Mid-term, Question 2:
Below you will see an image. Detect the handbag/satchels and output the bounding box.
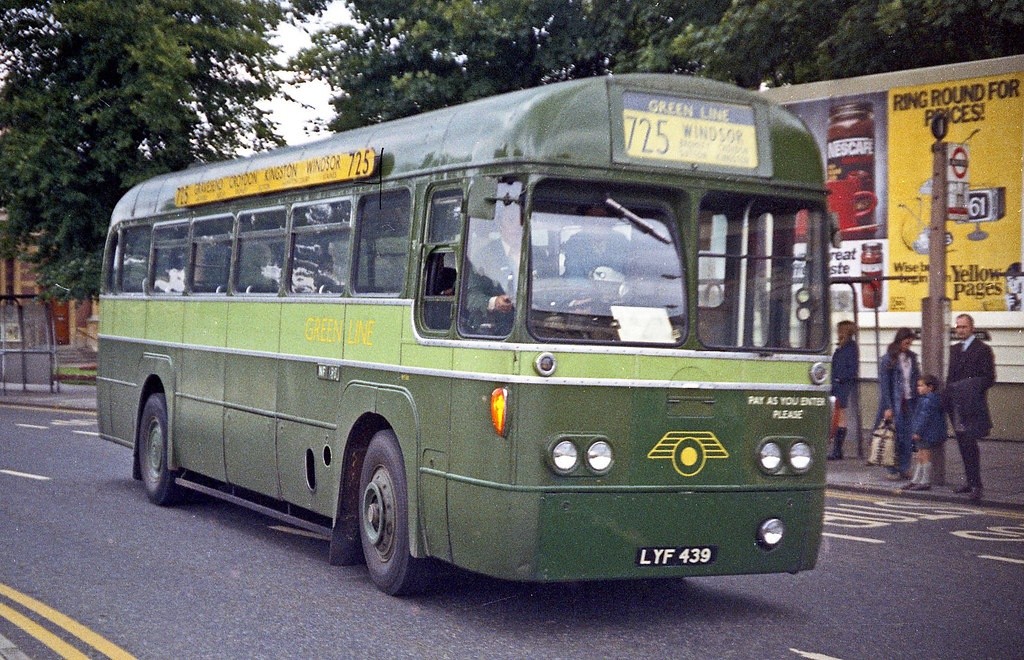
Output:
[867,416,896,467]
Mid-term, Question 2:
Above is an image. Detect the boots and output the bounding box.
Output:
[827,427,848,460]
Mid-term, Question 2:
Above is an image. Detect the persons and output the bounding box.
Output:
[563,203,629,277]
[899,375,948,489]
[827,321,859,460]
[877,328,920,487]
[944,314,995,501]
[463,201,545,331]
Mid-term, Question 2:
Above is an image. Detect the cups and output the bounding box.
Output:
[824,180,878,227]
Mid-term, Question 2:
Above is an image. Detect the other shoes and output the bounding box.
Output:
[885,469,912,481]
[910,483,930,491]
[900,482,916,490]
[953,485,970,494]
[969,487,982,500]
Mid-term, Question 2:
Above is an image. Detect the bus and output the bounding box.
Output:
[98,71,842,599]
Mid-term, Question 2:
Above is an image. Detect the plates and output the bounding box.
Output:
[839,224,877,240]
